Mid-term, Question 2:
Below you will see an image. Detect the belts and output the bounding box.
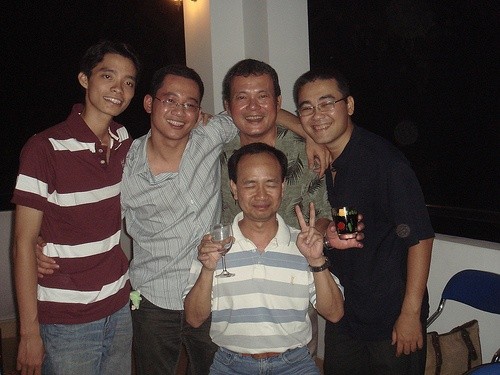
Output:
[239,350,294,360]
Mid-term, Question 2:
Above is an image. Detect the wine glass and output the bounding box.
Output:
[210,223,236,279]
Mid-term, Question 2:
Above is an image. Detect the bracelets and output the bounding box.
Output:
[322,236,333,250]
[308,259,328,272]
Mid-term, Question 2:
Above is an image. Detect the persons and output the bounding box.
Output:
[33,64,334,375]
[196,58,364,361]
[182,143,345,375]
[11,41,139,375]
[292,69,436,375]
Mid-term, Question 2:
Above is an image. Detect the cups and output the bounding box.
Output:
[330,205,359,240]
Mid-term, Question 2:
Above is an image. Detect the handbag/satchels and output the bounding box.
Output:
[423,320,482,375]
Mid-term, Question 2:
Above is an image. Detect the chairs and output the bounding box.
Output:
[421,269,500,375]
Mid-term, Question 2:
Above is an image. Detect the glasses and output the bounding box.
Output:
[295,97,345,117]
[152,96,201,111]
